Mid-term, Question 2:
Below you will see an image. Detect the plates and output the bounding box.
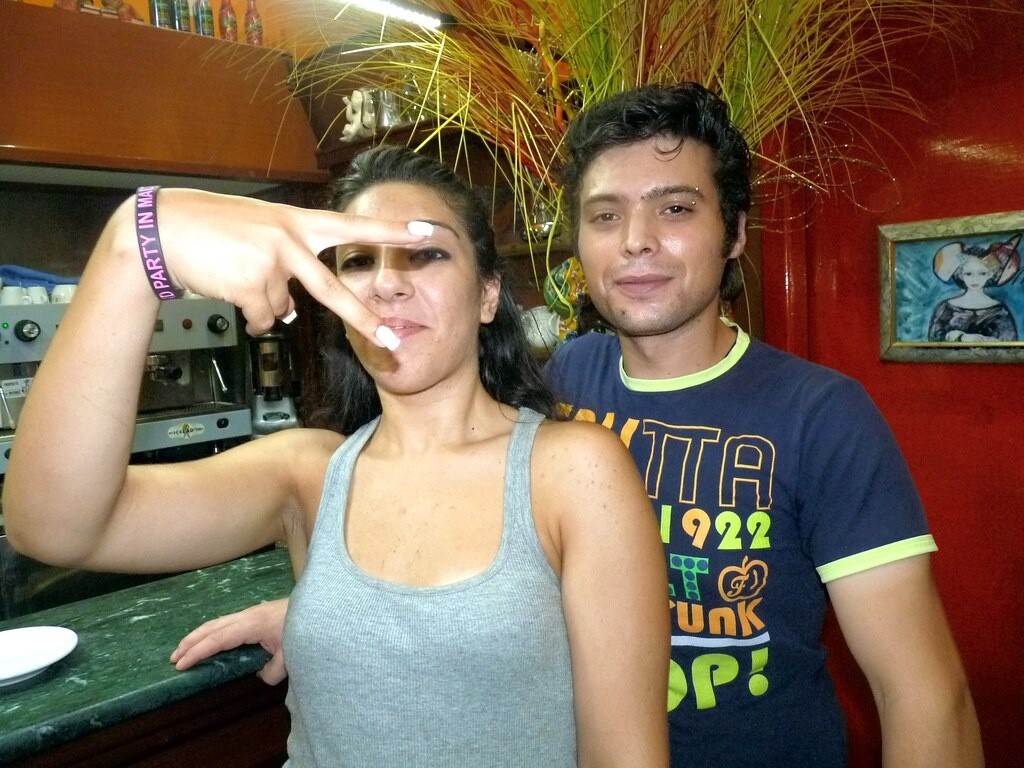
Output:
[0,626,77,686]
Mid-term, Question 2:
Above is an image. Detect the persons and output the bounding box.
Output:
[170,81,987,767]
[3,147,671,768]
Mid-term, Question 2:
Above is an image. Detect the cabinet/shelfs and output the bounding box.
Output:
[312,75,592,259]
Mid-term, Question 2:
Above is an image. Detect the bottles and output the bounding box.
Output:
[192,0,214,37]
[150,0,172,30]
[218,0,237,41]
[244,0,262,46]
[172,0,190,33]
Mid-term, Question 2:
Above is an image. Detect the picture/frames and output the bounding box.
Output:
[876,211,1023,364]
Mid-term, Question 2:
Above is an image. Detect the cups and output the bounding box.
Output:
[0,284,77,305]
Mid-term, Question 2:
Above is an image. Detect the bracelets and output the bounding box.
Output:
[136,184,184,301]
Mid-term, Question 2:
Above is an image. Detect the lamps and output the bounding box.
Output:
[337,0,459,30]
